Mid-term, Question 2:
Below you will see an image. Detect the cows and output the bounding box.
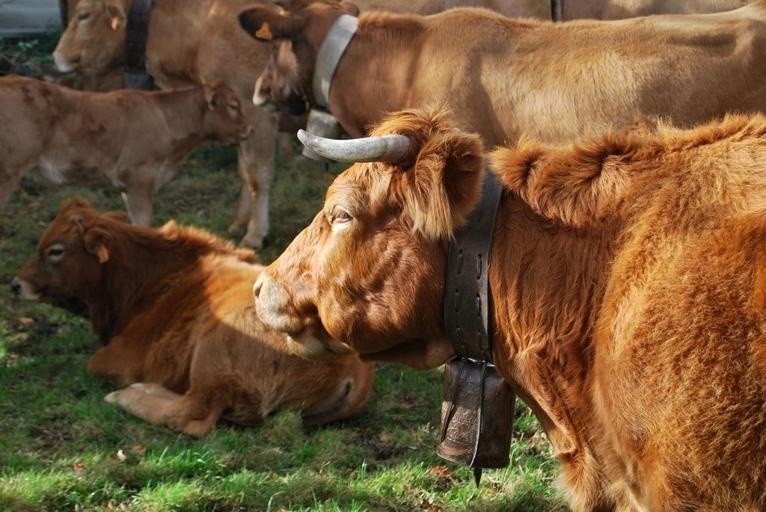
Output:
[0,2,765,512]
